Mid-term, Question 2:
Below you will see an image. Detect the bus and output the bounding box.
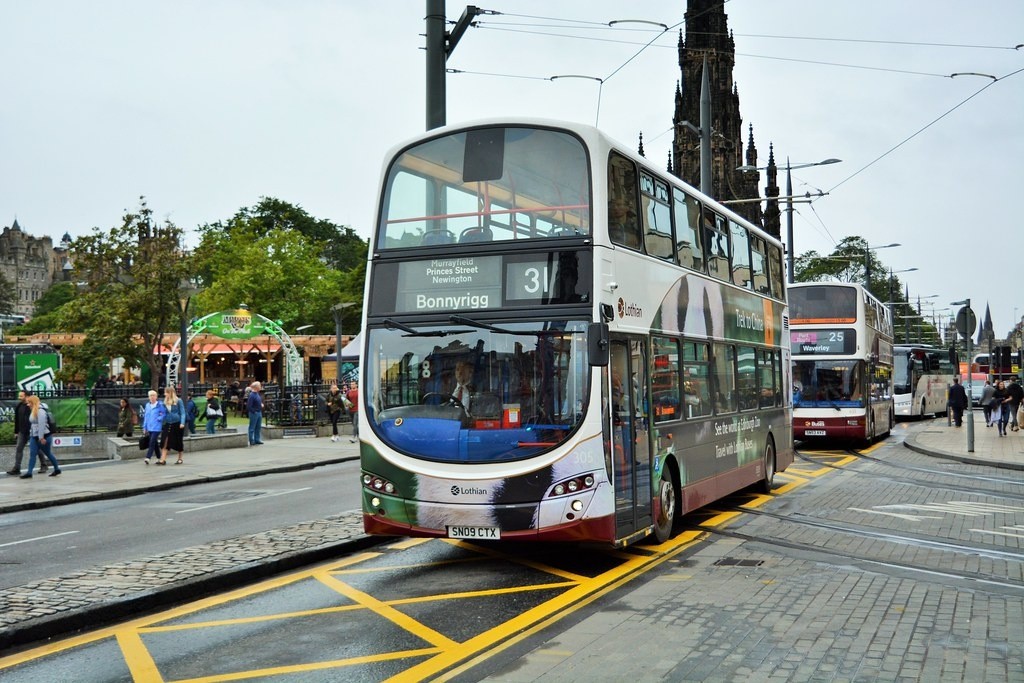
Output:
[788,278,894,448]
[355,115,794,552]
[893,343,961,420]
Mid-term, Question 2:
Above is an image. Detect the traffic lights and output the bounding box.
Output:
[928,353,941,371]
[992,346,1000,367]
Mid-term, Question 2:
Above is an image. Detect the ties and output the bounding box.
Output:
[458,387,463,401]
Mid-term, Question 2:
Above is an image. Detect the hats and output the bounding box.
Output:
[1010,376,1017,381]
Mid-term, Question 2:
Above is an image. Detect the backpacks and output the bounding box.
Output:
[191,405,199,416]
[46,412,57,434]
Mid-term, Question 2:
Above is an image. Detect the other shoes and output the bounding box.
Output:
[635,462,641,465]
[176,459,183,464]
[145,458,149,465]
[349,439,356,443]
[19,474,33,479]
[1009,424,1013,431]
[156,460,166,465]
[255,442,264,444]
[7,469,20,475]
[250,441,255,445]
[38,465,48,473]
[331,436,339,442]
[49,470,60,476]
[1013,428,1019,432]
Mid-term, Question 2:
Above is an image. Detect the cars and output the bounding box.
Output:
[959,351,1024,408]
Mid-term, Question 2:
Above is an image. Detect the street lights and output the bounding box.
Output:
[894,282,940,345]
[843,240,903,295]
[914,305,951,346]
[330,301,357,396]
[870,267,919,336]
[173,285,206,437]
[734,155,843,284]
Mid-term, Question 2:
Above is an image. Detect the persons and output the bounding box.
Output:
[746,384,781,406]
[7,390,61,479]
[978,376,1024,436]
[948,377,968,428]
[440,357,481,418]
[906,354,916,386]
[225,381,264,445]
[117,385,224,465]
[324,381,358,443]
[609,198,640,247]
[608,371,648,466]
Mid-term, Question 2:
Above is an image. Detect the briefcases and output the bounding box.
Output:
[139,433,150,450]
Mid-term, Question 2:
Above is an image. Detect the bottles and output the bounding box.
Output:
[340,397,354,408]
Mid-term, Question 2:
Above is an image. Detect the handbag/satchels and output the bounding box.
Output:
[207,402,224,416]
[132,411,138,424]
[178,399,192,422]
[324,397,330,412]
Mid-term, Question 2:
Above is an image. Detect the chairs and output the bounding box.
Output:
[401,222,580,242]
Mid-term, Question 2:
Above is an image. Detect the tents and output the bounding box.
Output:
[322,327,421,385]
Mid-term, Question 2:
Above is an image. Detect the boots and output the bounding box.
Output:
[1003,422,1007,435]
[998,424,1002,436]
